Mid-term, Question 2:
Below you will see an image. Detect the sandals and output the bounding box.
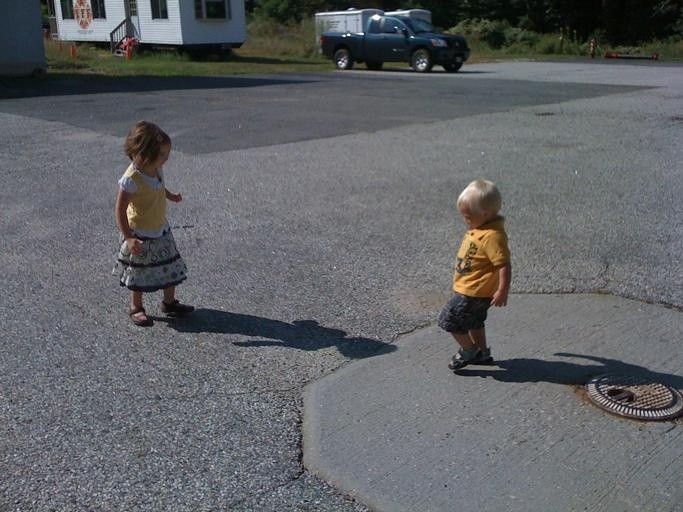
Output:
[447,347,482,370]
[471,347,492,365]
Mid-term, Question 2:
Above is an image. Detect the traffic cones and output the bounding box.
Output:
[57,43,65,53]
[71,41,79,59]
[125,38,133,60]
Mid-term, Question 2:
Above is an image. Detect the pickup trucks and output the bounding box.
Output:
[320,14,471,73]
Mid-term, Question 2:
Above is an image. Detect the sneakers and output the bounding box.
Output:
[161,299,194,313]
[128,305,152,326]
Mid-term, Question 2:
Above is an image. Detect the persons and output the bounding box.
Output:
[438,178,513,369]
[116,121,195,326]
